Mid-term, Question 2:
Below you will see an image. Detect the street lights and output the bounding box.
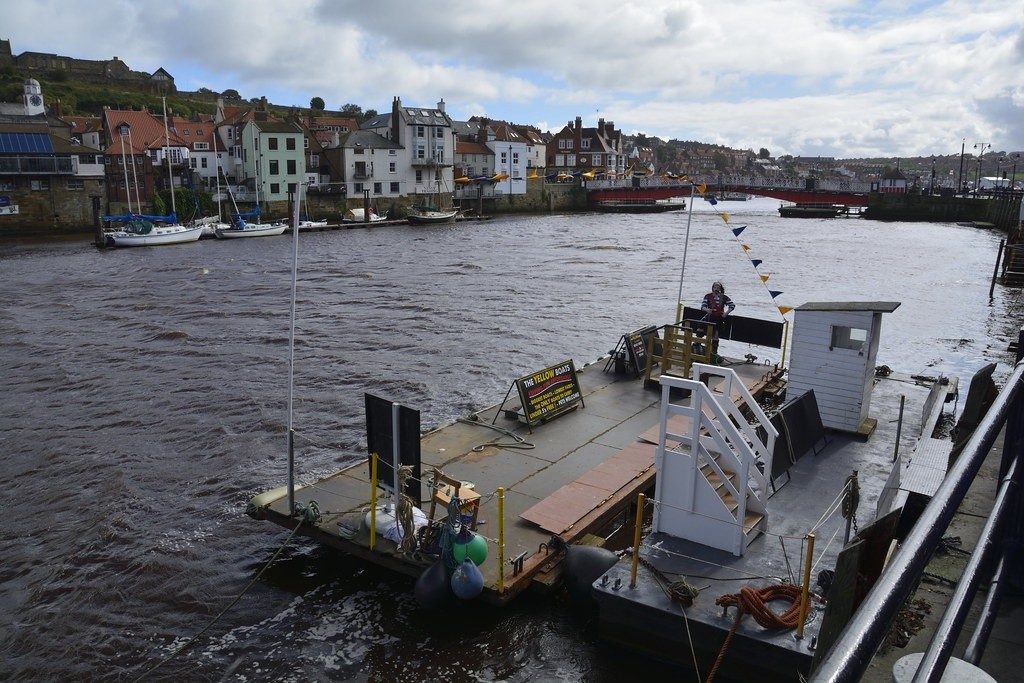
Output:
[1009,154,1020,200]
[994,157,1002,199]
[974,143,991,189]
[930,159,936,195]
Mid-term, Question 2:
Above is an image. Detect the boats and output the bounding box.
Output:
[343,208,387,223]
[778,201,839,218]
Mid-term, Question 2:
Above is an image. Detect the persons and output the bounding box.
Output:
[694,282,735,354]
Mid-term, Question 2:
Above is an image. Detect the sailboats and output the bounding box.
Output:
[407,163,458,223]
[98,96,287,247]
[287,182,328,231]
